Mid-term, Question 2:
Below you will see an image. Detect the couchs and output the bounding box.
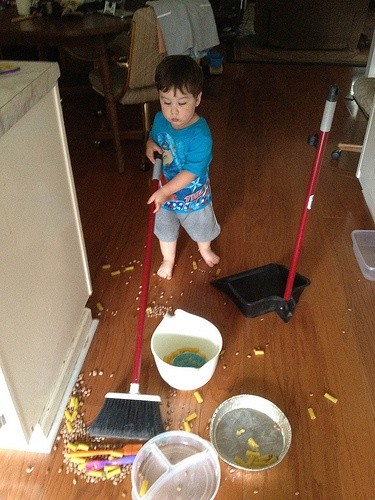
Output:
[253,0,371,49]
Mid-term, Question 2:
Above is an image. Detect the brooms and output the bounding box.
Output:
[85,150,165,441]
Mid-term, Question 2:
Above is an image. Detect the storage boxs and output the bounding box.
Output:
[351,230,375,281]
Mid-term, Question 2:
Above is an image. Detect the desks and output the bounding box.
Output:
[0,9,134,173]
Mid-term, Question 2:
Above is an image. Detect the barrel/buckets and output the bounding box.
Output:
[150,308,222,391]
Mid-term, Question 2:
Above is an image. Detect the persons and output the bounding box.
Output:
[145,56,220,280]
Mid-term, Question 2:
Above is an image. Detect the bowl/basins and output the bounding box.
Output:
[210,394,292,471]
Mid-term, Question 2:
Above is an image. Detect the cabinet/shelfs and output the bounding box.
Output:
[0,61,98,455]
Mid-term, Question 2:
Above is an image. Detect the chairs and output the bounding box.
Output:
[331,76,375,158]
[93,0,220,171]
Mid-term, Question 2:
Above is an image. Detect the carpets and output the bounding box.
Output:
[226,0,375,65]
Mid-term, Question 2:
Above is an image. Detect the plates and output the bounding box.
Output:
[131,431,221,500]
